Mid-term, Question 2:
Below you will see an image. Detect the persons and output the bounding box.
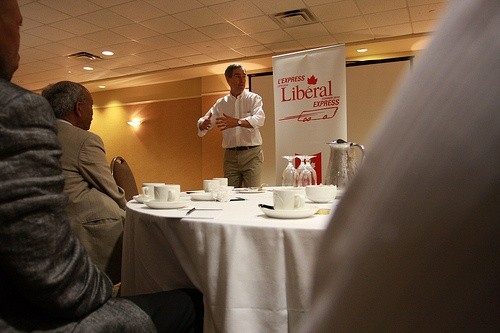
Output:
[41,80,129,285]
[303,1,500,333]
[0,0,206,333]
[197,64,265,187]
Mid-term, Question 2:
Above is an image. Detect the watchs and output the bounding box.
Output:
[237,119,242,126]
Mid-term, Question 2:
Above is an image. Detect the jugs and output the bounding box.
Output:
[324,138,366,190]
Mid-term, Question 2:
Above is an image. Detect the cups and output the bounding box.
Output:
[154,184,181,202]
[273,187,306,210]
[202,177,235,201]
[140,182,165,197]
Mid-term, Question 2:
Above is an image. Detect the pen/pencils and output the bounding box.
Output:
[186,207,196,215]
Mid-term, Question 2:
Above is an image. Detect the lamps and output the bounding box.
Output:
[127,117,146,127]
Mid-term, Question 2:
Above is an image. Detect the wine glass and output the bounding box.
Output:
[281,155,318,187]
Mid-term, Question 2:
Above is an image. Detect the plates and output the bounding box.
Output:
[261,204,322,218]
[190,191,217,200]
[133,194,191,208]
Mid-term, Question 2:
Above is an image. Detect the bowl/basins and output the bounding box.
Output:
[306,184,337,203]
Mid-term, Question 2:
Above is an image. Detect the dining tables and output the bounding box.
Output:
[120,185,348,333]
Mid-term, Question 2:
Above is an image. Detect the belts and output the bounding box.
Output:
[228,146,257,151]
